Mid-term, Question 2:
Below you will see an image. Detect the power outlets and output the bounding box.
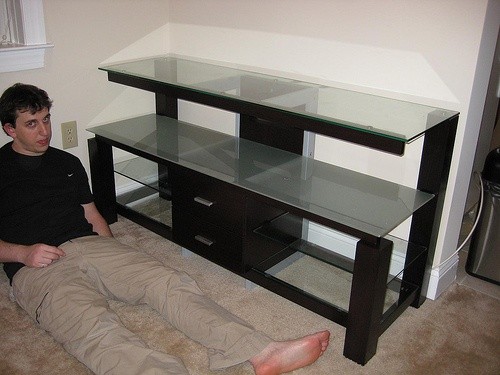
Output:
[60,120,79,150]
[469,158,484,187]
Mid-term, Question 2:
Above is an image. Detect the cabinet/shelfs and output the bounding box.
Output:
[84,54,461,366]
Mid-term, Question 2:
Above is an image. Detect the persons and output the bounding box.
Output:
[0,82,330,375]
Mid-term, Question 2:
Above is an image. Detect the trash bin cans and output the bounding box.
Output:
[465,148,500,286]
